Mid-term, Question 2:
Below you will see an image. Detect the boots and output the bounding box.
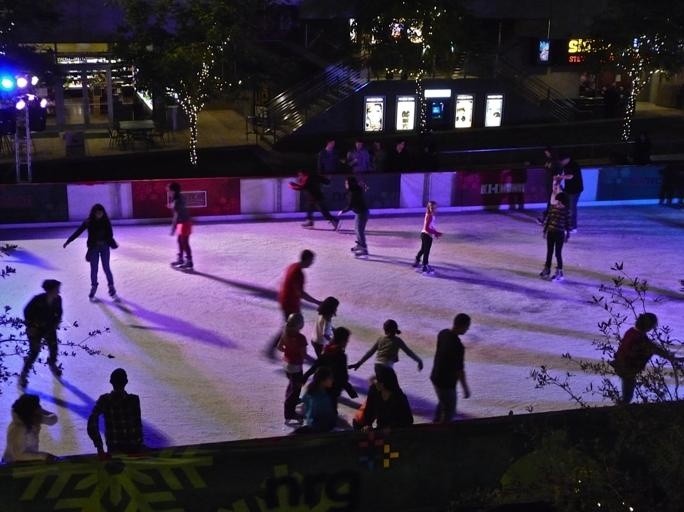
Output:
[413,259,432,272]
[285,408,303,419]
[301,221,313,226]
[332,219,339,231]
[539,265,564,280]
[351,240,367,257]
[170,252,183,266]
[89,282,98,299]
[107,282,115,296]
[181,254,193,269]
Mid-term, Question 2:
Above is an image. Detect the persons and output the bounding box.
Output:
[1,391,59,463]
[86,367,144,455]
[266,249,323,359]
[538,148,585,280]
[338,177,371,256]
[365,104,382,132]
[63,203,117,298]
[165,181,193,269]
[414,200,443,272]
[315,139,406,173]
[17,278,64,388]
[613,312,675,403]
[288,169,339,230]
[275,295,471,432]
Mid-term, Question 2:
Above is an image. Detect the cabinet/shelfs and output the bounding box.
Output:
[56,52,139,96]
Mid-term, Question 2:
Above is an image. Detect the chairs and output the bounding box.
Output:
[107,115,167,151]
[0,119,36,156]
